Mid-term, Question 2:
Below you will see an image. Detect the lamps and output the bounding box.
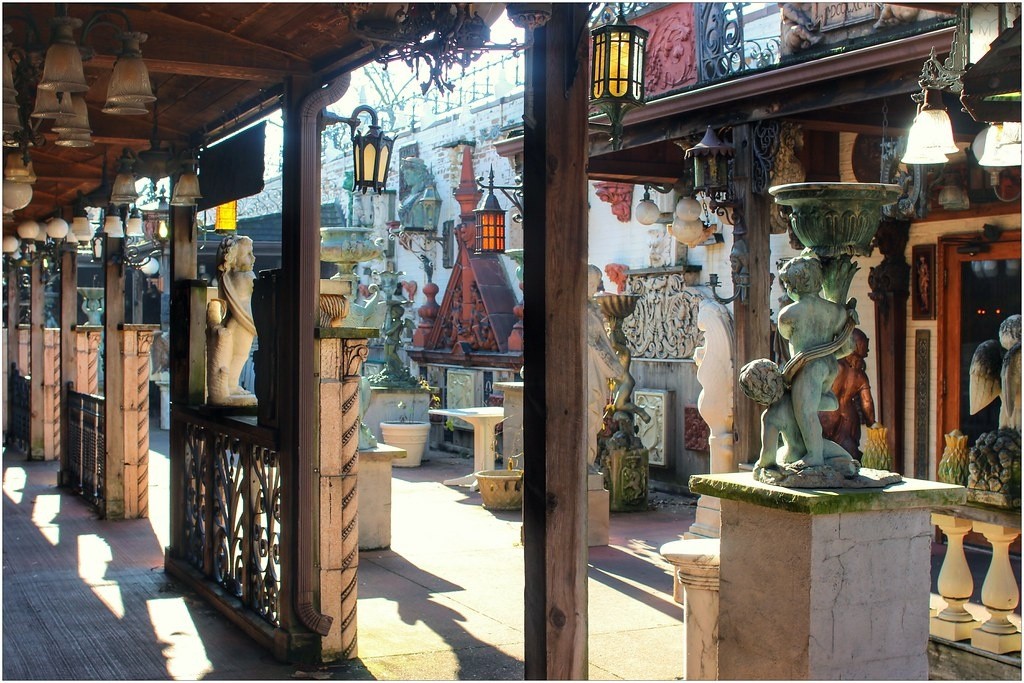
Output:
[418,160,458,269]
[322,98,400,196]
[472,161,525,258]
[684,113,745,227]
[898,39,1021,166]
[565,2,653,154]
[3,3,241,296]
[633,178,722,246]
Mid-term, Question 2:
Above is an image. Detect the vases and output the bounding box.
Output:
[473,468,523,509]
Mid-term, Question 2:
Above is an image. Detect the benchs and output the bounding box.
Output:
[358,443,408,550]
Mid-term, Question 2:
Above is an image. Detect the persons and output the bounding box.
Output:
[207,236,256,395]
[331,274,386,430]
[586,265,625,467]
[781,2,823,52]
[738,257,860,482]
[82,297,104,326]
[872,3,919,29]
[385,305,404,368]
[609,316,652,424]
[398,157,440,230]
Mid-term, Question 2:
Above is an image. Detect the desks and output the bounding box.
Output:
[661,538,720,681]
[426,404,508,494]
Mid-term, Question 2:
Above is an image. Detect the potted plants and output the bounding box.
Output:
[379,378,456,469]
